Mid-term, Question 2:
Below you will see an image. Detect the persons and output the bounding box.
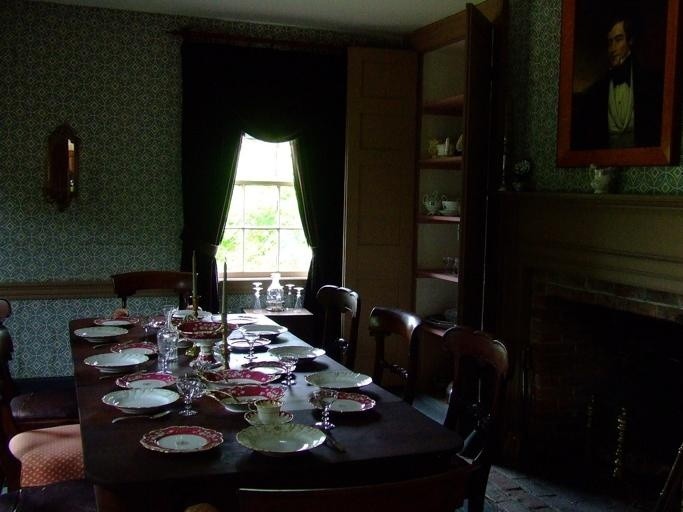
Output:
[584,20,659,148]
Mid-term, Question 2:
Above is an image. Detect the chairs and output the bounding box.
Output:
[311,282,362,372]
[231,466,471,511]
[0,296,80,432]
[0,328,96,511]
[366,304,422,410]
[437,321,510,511]
[110,269,193,310]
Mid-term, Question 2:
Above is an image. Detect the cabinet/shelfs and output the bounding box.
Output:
[338,0,509,403]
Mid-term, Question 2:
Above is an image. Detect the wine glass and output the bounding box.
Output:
[248,271,307,314]
[312,372,341,427]
[424,254,459,329]
[242,327,260,359]
[177,376,196,416]
[157,336,173,375]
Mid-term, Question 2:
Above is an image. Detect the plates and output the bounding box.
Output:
[239,325,289,338]
[173,309,206,319]
[205,384,288,413]
[207,370,281,385]
[101,388,181,414]
[115,372,178,390]
[307,390,377,414]
[245,410,294,426]
[94,316,140,327]
[241,361,297,375]
[74,327,128,342]
[139,426,225,456]
[234,427,328,460]
[267,346,326,367]
[211,313,259,326]
[226,337,272,350]
[111,341,156,355]
[83,353,149,374]
[304,370,373,391]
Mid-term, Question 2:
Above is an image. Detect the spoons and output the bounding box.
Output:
[112,410,171,424]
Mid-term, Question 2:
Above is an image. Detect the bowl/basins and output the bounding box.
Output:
[420,196,462,218]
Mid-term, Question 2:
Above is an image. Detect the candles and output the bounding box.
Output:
[221,261,229,313]
[189,249,199,298]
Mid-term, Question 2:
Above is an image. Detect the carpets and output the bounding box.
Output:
[457,459,558,512]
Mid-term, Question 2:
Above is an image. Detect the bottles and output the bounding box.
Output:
[156,307,178,361]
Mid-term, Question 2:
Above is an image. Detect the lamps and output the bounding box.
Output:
[42,121,83,214]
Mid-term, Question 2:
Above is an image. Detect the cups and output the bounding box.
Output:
[185,367,204,398]
[254,399,282,425]
[437,144,454,156]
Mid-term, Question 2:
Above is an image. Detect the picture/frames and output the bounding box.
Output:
[554,1,682,169]
